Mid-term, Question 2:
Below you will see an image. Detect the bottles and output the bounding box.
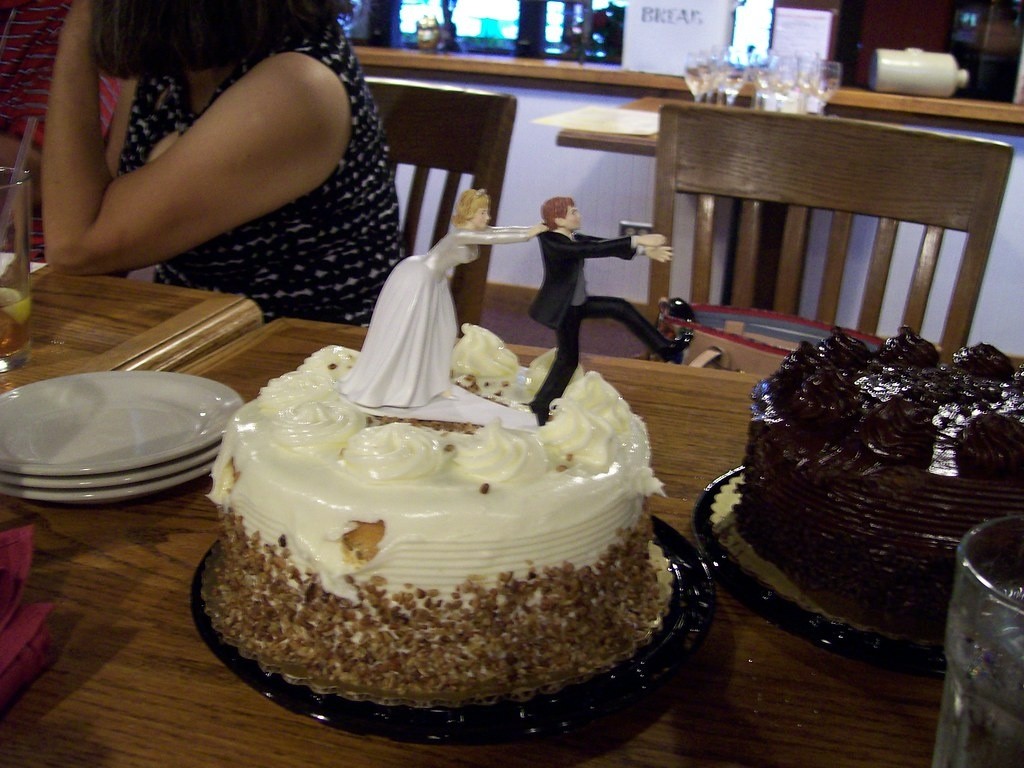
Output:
[871,48,970,98]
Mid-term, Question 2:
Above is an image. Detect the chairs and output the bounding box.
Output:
[364,76,520,337]
[641,100,1015,365]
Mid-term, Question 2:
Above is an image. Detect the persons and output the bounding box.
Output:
[528,196,694,428]
[42,0,401,331]
[0,0,124,262]
[339,188,549,408]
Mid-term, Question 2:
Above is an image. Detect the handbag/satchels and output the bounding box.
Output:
[641,296,884,375]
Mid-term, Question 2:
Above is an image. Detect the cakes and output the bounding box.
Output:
[739,325,1024,627]
[207,322,666,703]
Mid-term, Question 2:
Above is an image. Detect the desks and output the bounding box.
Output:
[351,45,1024,370]
[0,271,944,768]
[555,94,904,318]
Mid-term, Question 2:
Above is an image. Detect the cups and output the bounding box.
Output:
[0,167,37,373]
[931,516,1024,768]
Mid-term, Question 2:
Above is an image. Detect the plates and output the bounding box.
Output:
[189,514,716,746]
[0,371,246,506]
[690,465,951,682]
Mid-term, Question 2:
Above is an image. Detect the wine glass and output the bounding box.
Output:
[685,46,844,116]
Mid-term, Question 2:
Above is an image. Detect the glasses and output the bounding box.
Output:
[666,296,695,322]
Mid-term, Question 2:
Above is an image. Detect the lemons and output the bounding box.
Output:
[0,287,31,324]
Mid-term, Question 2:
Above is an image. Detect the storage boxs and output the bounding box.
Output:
[620,0,745,78]
